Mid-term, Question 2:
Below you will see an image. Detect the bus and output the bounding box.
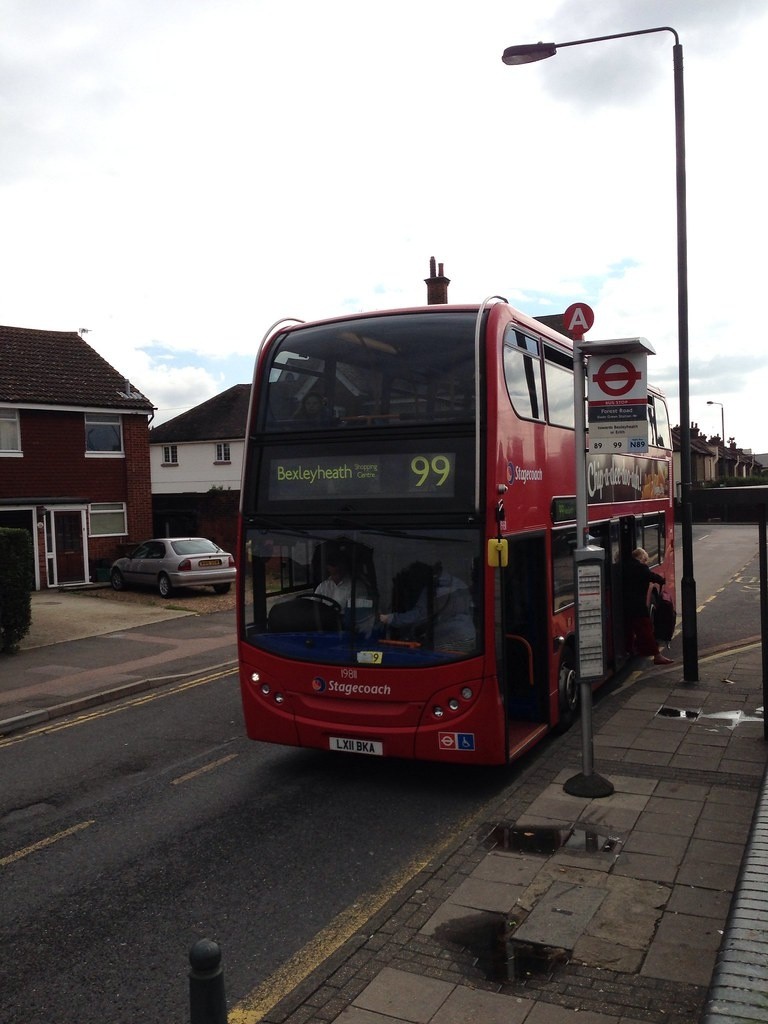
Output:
[236,294,676,768]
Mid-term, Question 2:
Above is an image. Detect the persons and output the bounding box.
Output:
[289,391,338,432]
[279,373,297,386]
[309,554,373,631]
[380,554,477,653]
[622,548,675,665]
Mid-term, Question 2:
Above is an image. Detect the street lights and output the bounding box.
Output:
[707,401,727,486]
[503,26,702,687]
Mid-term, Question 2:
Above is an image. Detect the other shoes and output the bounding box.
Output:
[654,656,674,665]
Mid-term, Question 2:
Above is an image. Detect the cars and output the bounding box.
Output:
[110,536,236,598]
